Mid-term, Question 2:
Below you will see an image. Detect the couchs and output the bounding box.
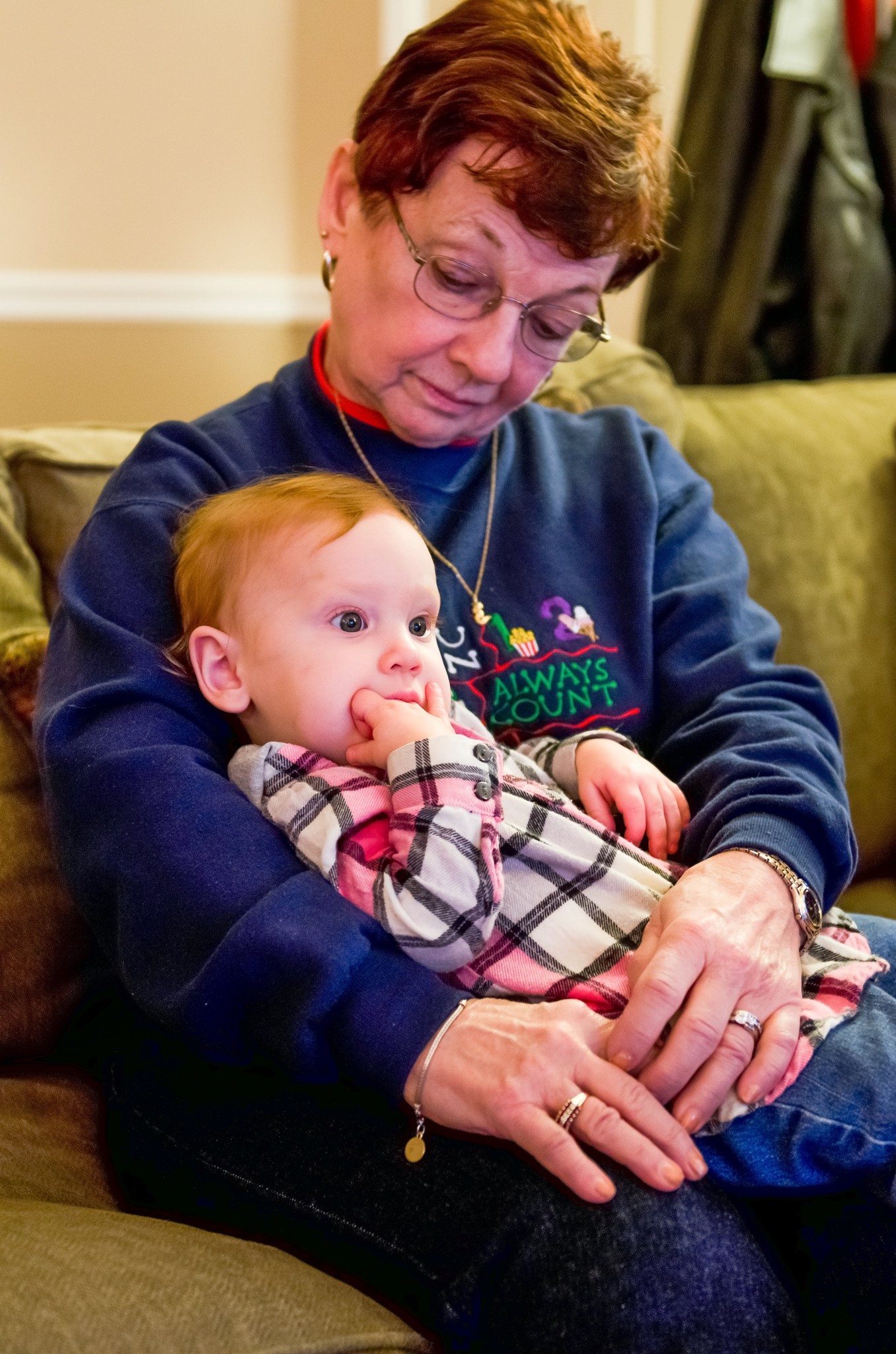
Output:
[0,374,896,1354]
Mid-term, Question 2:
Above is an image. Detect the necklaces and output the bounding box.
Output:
[332,385,498,626]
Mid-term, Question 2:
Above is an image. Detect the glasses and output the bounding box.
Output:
[386,189,608,363]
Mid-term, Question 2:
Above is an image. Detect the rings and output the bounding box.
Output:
[728,1009,763,1043]
[555,1091,589,1132]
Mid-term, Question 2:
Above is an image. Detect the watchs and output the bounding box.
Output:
[721,847,822,957]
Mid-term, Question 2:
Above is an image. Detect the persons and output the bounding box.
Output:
[160,468,896,1190]
[35,0,895,1354]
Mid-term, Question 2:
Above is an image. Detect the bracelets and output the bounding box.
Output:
[404,998,466,1163]
